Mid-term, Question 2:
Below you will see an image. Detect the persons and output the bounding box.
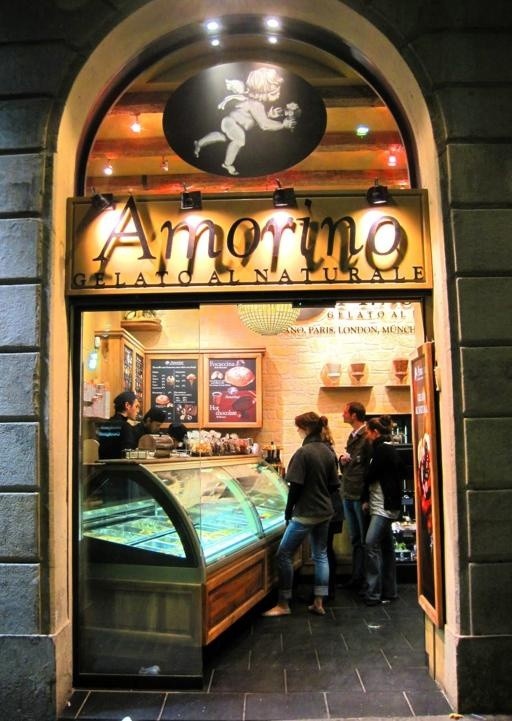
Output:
[262,410,335,616]
[338,402,373,597]
[168,421,187,449]
[98,392,144,502]
[299,418,346,603]
[192,67,297,178]
[356,414,403,608]
[132,406,166,451]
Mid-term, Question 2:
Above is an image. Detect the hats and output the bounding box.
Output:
[169,423,187,440]
[113,392,135,404]
[148,408,166,421]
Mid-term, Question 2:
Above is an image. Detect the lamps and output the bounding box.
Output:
[179,185,202,211]
[87,185,111,211]
[366,182,389,205]
[235,302,297,337]
[272,184,298,208]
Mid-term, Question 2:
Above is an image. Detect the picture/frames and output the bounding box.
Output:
[407,342,444,631]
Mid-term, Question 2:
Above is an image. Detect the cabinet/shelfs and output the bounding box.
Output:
[362,414,417,568]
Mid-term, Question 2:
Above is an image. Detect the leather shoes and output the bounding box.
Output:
[355,591,398,605]
[308,605,325,614]
[262,606,291,616]
